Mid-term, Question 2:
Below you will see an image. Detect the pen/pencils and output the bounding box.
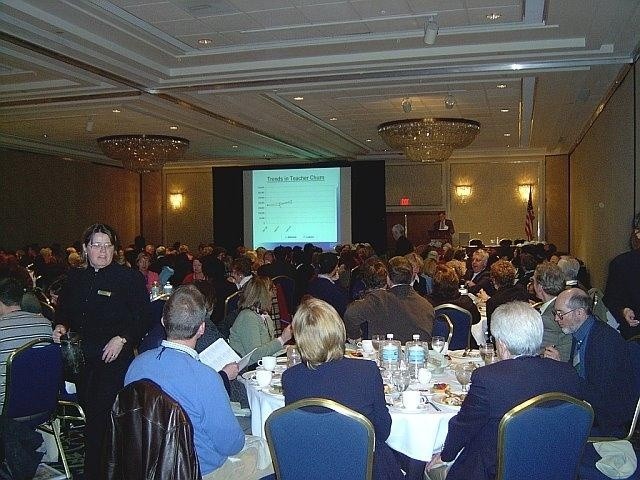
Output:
[280,319,290,324]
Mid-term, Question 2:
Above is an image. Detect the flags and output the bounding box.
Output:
[524,185,535,240]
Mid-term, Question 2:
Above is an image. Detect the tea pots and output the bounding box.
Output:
[59,331,87,375]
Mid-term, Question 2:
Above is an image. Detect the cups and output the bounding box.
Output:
[251,339,449,410]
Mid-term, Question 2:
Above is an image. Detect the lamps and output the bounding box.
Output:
[456,184,471,203]
[422,16,438,44]
[170,189,183,210]
[518,184,535,204]
[377,118,480,162]
[98,135,189,173]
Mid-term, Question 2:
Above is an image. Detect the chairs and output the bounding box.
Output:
[514,239,526,245]
[499,239,512,245]
[469,238,483,246]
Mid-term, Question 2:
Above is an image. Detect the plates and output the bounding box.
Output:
[241,347,483,414]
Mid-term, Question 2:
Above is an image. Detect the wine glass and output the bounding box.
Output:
[287,335,496,409]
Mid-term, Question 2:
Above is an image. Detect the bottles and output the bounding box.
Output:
[459,285,468,296]
[152,279,173,300]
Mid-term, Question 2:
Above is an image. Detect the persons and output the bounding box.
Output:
[282,295,405,480]
[124,286,275,480]
[425,301,585,480]
[0,277,55,480]
[51,223,151,480]
[545,288,640,438]
[0,210,640,386]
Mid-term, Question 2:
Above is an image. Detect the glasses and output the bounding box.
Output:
[90,244,112,248]
[552,308,578,320]
